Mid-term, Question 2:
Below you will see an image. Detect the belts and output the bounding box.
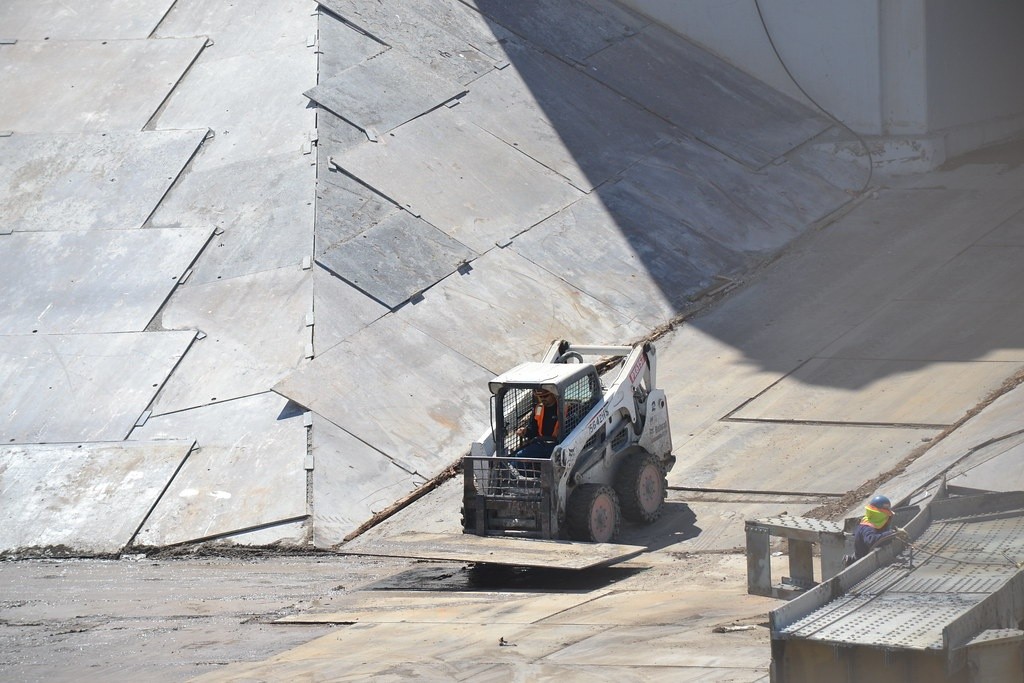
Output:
[536,441,557,444]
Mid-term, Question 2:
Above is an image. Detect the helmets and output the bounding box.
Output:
[864,495,896,515]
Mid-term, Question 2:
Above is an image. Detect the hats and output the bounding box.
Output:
[534,388,551,396]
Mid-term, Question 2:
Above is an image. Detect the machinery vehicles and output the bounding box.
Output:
[455,339,677,575]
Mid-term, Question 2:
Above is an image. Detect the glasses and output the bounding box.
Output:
[537,393,551,398]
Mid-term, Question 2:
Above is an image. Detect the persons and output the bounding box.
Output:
[510,386,579,478]
[846,495,910,567]
